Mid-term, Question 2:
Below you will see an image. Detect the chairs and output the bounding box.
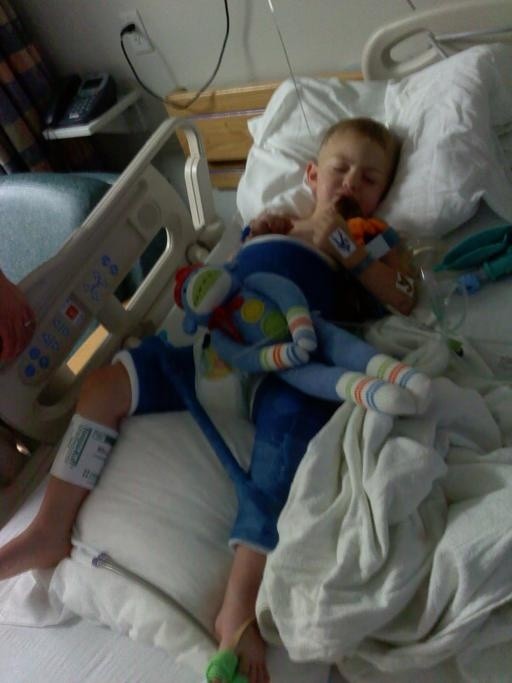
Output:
[1,169,164,345]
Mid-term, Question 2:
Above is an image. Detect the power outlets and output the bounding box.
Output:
[117,7,154,57]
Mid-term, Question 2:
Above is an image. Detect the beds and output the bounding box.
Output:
[1,0,511,683]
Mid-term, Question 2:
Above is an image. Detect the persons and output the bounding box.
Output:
[0,118,422,683]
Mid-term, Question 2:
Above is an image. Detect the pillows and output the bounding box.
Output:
[233,39,511,228]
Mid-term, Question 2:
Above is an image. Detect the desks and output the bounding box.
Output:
[41,83,145,141]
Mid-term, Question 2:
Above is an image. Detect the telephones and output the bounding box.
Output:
[43,71,117,128]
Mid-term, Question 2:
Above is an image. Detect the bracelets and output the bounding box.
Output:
[350,254,374,281]
